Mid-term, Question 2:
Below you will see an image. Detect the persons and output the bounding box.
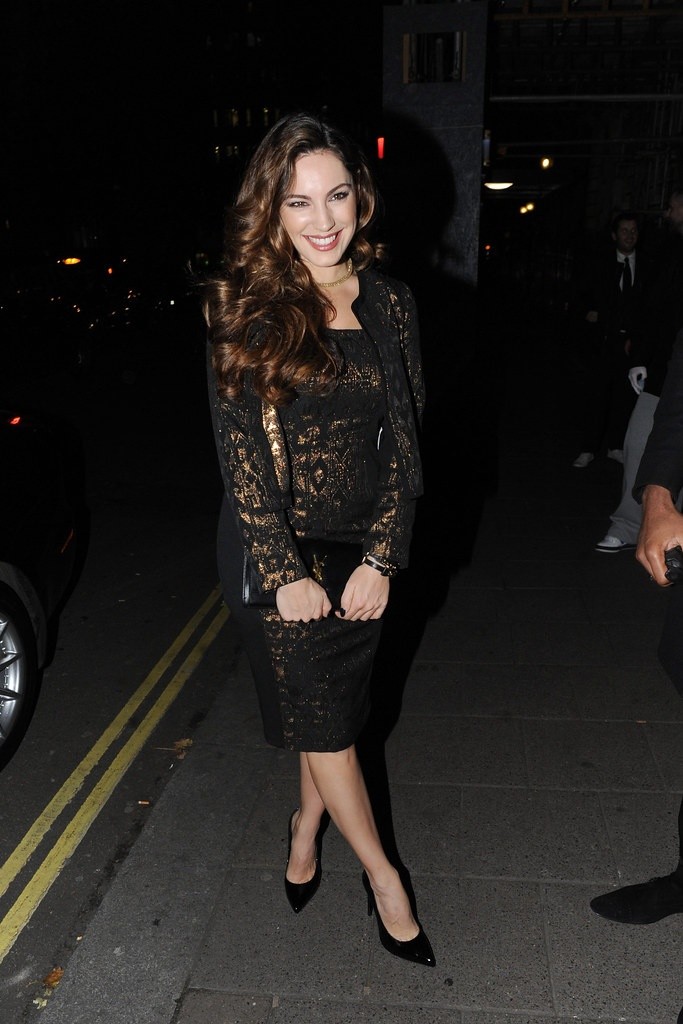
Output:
[580,188,683,925]
[202,113,438,966]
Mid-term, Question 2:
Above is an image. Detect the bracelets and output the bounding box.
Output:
[361,557,393,578]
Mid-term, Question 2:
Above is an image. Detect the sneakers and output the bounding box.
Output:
[594,533,639,554]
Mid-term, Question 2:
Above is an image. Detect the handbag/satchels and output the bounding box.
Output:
[240,534,363,610]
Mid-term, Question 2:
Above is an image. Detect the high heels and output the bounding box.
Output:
[284,808,331,914]
[362,869,438,967]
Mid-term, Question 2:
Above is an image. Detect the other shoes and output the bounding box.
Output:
[590,868,683,926]
[607,447,626,464]
[574,452,594,468]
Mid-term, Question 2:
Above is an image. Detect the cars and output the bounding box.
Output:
[0,409,89,771]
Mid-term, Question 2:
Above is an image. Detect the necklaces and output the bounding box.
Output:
[315,258,353,288]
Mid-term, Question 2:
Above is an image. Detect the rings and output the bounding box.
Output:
[649,576,653,581]
